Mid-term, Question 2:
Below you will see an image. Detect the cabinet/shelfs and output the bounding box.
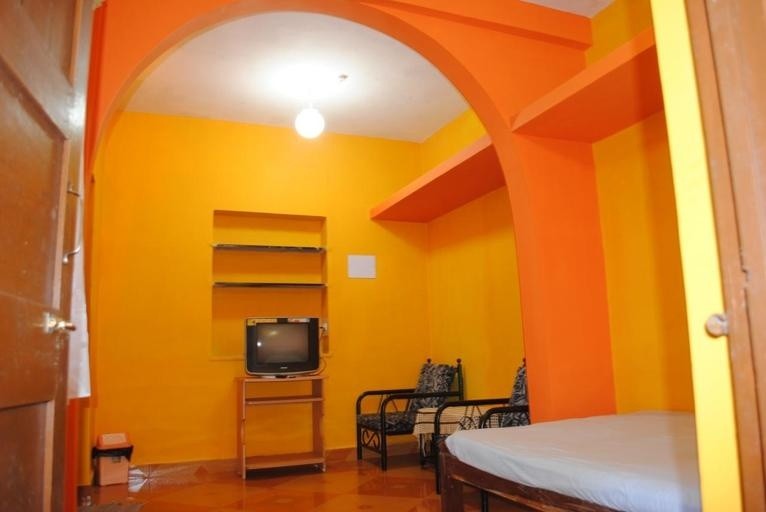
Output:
[213,242,325,288]
[236,377,326,480]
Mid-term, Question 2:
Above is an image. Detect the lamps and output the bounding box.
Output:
[296,105,324,139]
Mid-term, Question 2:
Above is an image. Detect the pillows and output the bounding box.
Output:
[412,363,454,412]
[501,360,529,426]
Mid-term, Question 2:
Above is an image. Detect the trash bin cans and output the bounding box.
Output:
[94,433,132,487]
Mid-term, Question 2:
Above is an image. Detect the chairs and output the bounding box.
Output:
[356,358,463,471]
[434,398,529,512]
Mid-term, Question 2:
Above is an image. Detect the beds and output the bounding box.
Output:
[438,411,701,512]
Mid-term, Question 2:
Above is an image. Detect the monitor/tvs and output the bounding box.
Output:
[244,317,319,378]
